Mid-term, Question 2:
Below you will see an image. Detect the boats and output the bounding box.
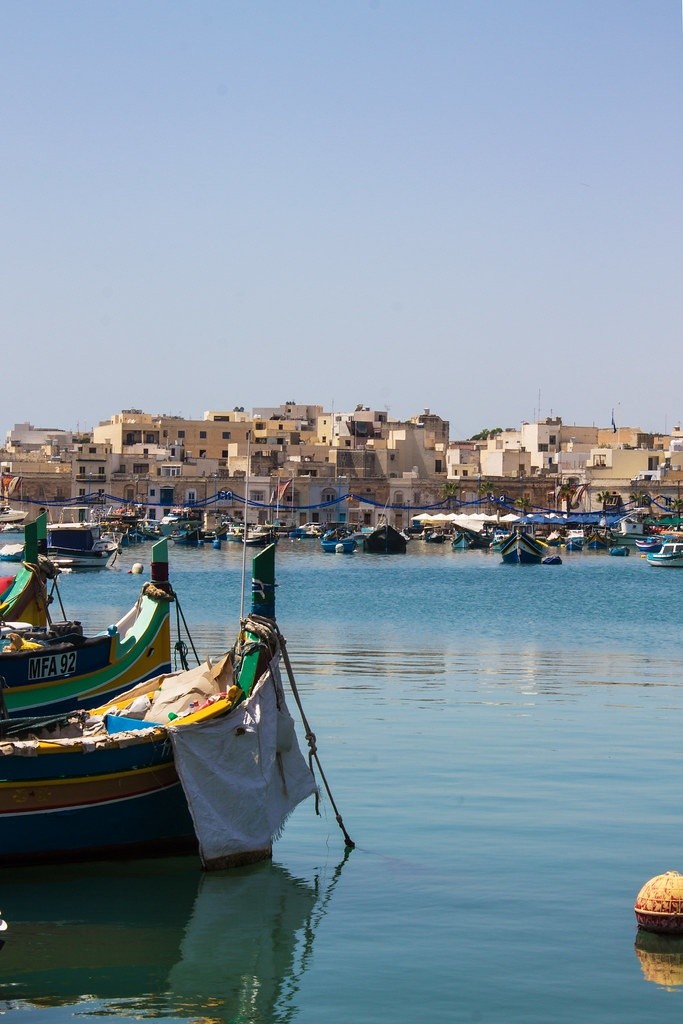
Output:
[608,545,630,557]
[0,544,283,855]
[0,538,175,722]
[0,512,53,630]
[646,543,683,566]
[635,535,681,553]
[0,505,29,524]
[45,522,119,568]
[104,467,612,565]
